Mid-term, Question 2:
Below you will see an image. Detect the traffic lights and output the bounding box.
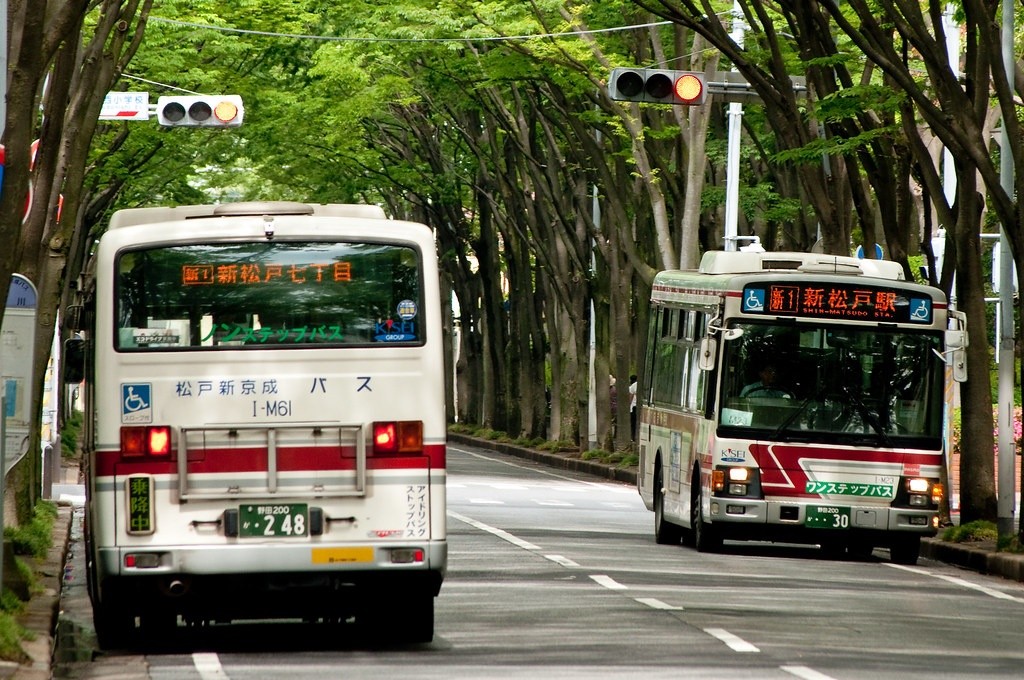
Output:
[609,66,709,105]
[156,94,244,126]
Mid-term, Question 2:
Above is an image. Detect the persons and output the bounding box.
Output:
[738,365,792,399]
[609,375,618,437]
[629,375,637,442]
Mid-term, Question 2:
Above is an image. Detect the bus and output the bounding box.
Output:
[61,203,448,651]
[636,235,970,566]
